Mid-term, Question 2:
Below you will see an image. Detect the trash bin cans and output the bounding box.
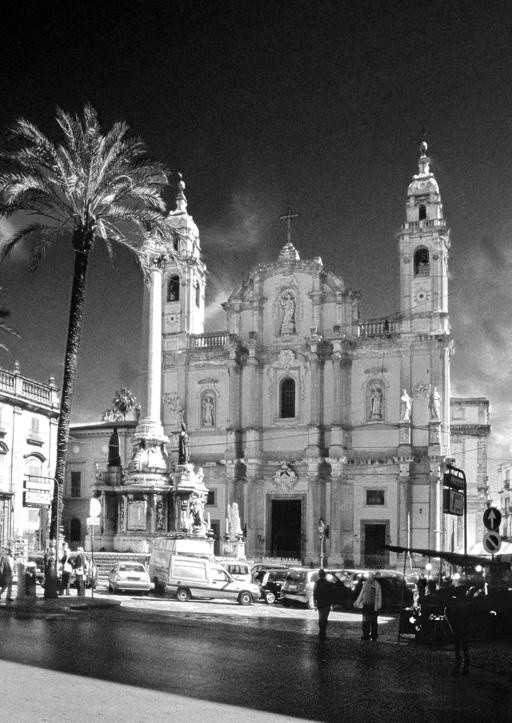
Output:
[16,562,37,601]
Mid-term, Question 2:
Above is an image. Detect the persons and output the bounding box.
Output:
[0,546,15,603]
[408,573,472,674]
[351,569,384,642]
[28,544,55,575]
[71,545,87,595]
[311,569,337,637]
[57,541,72,597]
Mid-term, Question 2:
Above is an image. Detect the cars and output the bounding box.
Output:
[250,564,416,609]
[108,561,150,596]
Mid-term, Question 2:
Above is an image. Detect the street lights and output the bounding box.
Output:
[318,521,325,570]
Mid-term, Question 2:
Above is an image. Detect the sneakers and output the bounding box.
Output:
[361,633,379,641]
[6,598,14,602]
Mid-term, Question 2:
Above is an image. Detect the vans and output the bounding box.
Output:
[149,538,261,606]
